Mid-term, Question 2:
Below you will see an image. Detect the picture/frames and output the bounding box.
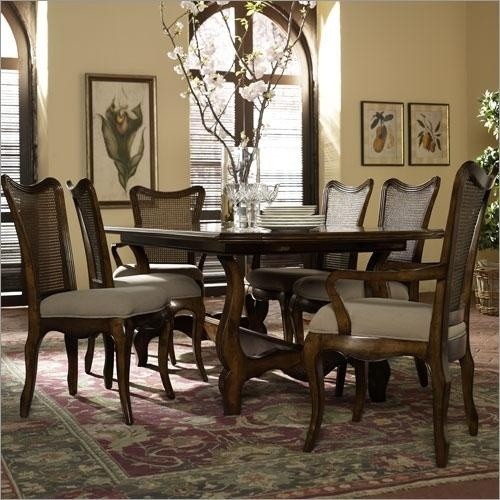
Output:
[407,102,451,166]
[360,100,405,167]
[83,72,159,210]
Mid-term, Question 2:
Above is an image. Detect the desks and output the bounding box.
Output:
[103,224,449,418]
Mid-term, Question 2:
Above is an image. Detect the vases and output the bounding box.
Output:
[220,146,260,232]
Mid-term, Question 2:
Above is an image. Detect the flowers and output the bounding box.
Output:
[157,0,317,207]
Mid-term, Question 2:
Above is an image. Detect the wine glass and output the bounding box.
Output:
[223,182,279,234]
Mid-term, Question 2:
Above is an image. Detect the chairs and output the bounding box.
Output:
[238,176,444,397]
[298,160,498,467]
[109,184,209,368]
[62,178,211,386]
[1,172,174,425]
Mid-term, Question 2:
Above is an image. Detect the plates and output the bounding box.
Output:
[257,204,327,232]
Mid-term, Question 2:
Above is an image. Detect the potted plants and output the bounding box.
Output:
[473,88,500,318]
